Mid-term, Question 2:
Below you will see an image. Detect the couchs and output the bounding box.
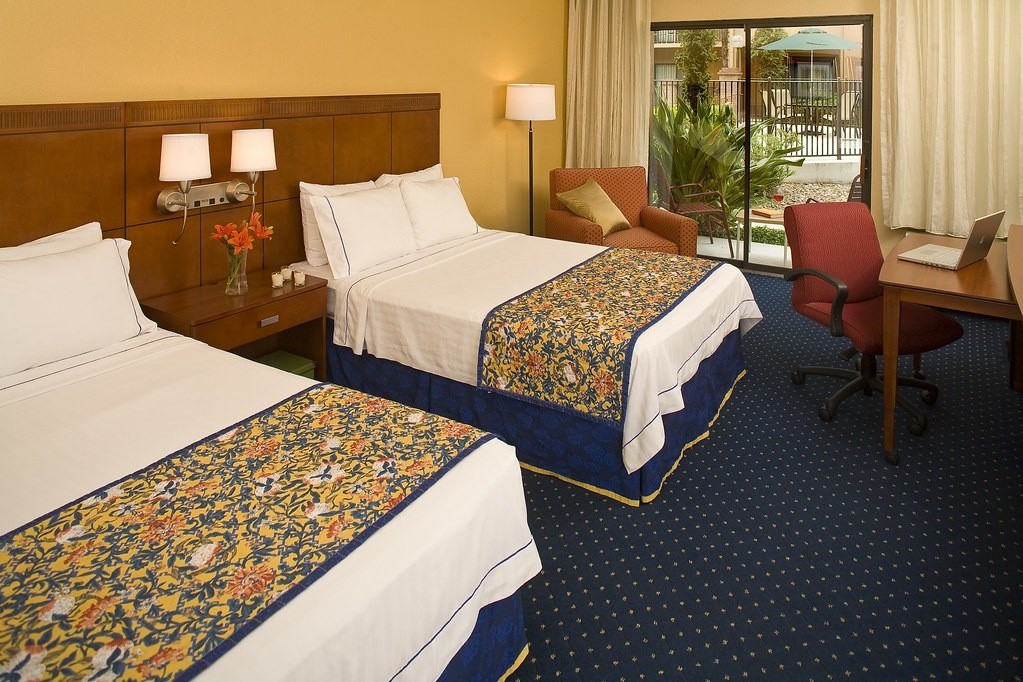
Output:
[542,168,700,256]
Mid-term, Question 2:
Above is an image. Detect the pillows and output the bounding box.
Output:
[0,236,157,375]
[378,163,445,239]
[555,176,632,239]
[0,222,103,261]
[299,179,377,267]
[310,182,411,280]
[400,177,479,248]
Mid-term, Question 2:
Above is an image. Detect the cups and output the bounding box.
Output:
[294,270,306,286]
[271,272,284,289]
[280,265,293,281]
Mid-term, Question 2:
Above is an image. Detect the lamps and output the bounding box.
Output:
[505,83,555,236]
[227,129,278,220]
[157,133,209,245]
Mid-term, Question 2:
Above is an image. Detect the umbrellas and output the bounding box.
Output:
[756,28,864,122]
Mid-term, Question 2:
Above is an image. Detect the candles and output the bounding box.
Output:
[271,271,284,289]
[292,271,306,287]
[281,265,292,282]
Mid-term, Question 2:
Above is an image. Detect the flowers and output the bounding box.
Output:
[209,211,274,292]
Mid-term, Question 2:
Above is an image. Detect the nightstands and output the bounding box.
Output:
[140,270,329,382]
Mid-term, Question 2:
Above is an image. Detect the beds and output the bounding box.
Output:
[0,221,543,682]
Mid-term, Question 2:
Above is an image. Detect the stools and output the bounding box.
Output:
[736,206,790,266]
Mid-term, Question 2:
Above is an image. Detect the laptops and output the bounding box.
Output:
[898,210,1005,271]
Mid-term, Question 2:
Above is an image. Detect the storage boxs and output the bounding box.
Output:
[252,348,317,381]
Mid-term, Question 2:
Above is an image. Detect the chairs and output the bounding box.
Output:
[652,157,734,257]
[807,173,862,204]
[782,200,962,439]
[758,87,862,137]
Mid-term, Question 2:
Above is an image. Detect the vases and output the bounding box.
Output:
[225,249,249,296]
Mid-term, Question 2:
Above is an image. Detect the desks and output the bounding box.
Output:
[784,103,837,136]
[878,224,1023,465]
